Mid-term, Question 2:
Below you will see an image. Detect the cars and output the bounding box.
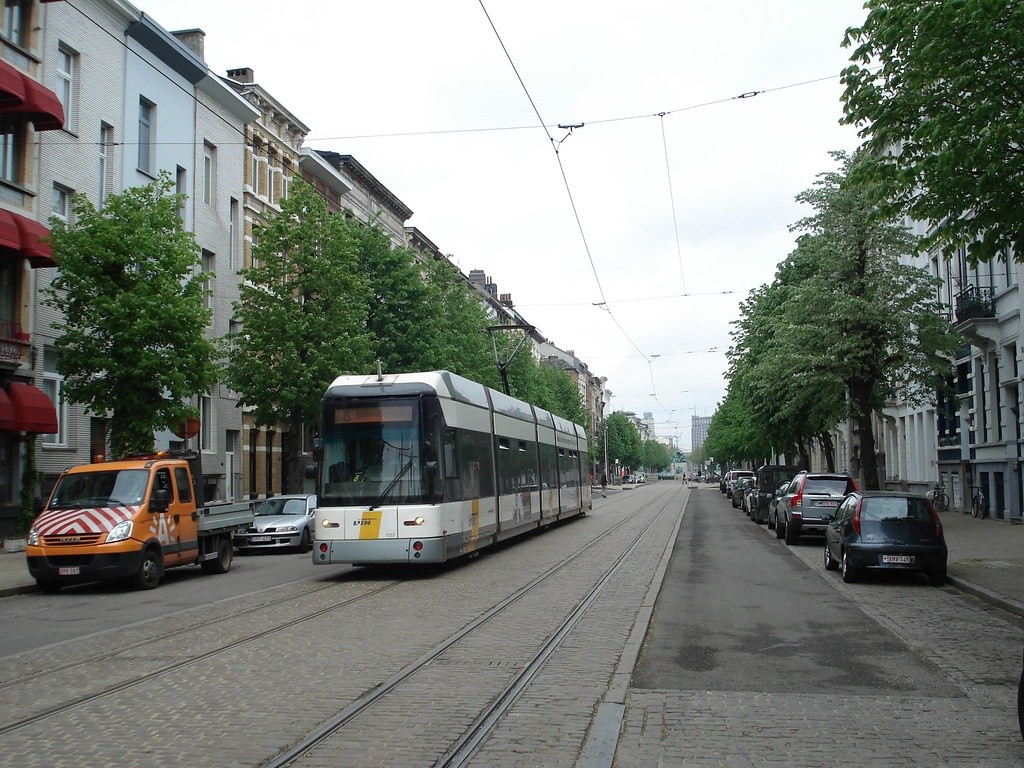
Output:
[825,488,950,587]
[622,474,647,485]
[234,493,319,555]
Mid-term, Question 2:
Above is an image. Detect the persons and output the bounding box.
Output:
[600,469,609,499]
[697,468,702,483]
[681,471,689,485]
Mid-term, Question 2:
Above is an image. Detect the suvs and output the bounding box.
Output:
[720,462,861,546]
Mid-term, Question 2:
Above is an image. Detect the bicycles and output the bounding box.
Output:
[933,483,952,513]
[970,484,988,521]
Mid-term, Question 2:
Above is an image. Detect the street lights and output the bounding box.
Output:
[604,426,609,486]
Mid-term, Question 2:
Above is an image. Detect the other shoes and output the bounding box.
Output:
[602,495,607,498]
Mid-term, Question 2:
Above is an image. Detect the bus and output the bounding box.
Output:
[312,325,593,566]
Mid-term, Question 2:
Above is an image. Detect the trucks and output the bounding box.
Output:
[25,449,257,589]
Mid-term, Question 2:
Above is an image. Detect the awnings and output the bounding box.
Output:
[0,381,59,434]
[0,208,62,269]
[0,58,65,135]
